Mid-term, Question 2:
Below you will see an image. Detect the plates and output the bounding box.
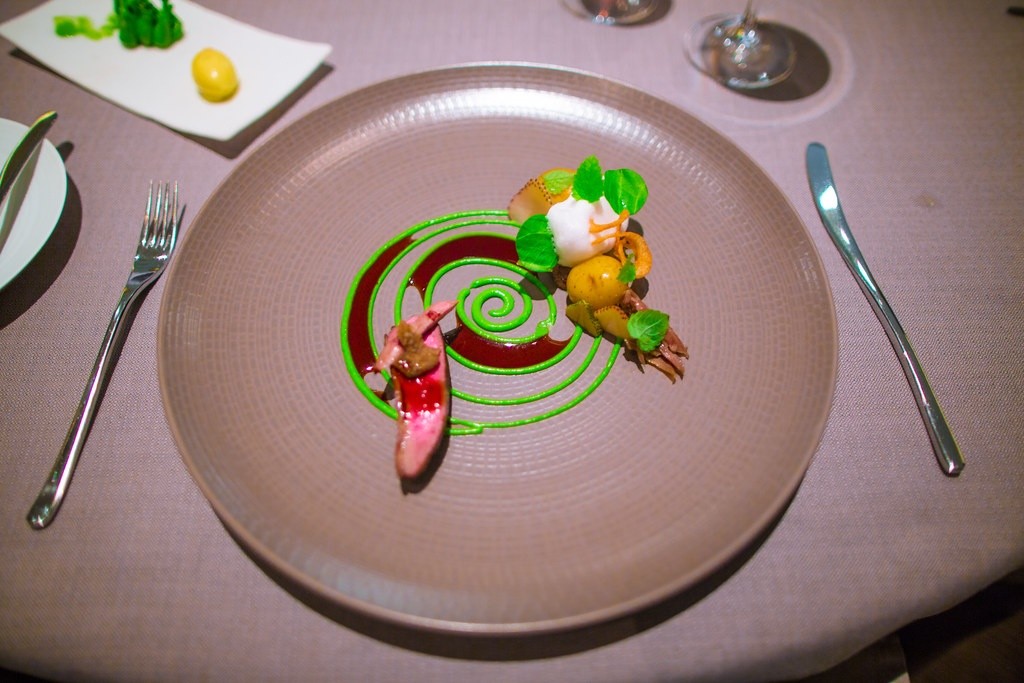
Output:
[0,117,69,299]
[156,59,844,635]
[0,1,337,143]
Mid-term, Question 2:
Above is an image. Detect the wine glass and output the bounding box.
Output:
[683,0,798,91]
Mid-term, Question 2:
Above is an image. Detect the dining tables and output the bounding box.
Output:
[0,1,1024,681]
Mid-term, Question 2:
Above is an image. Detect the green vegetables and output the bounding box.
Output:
[115,0,183,49]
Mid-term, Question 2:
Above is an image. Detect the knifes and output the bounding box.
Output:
[803,140,964,484]
[0,108,60,211]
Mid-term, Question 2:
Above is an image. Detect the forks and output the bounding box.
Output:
[28,179,180,529]
